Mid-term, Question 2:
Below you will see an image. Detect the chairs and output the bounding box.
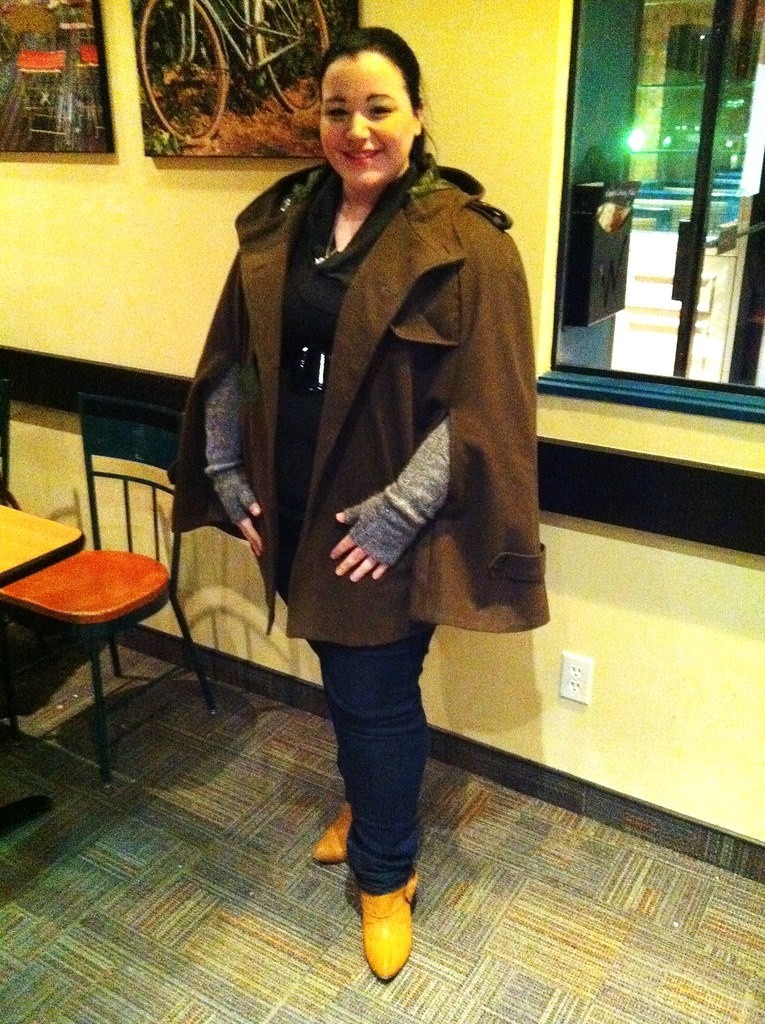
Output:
[0,393,217,786]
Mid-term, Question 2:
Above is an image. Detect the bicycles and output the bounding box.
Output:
[136,0,331,145]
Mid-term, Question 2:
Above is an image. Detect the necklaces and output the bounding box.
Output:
[314,222,341,264]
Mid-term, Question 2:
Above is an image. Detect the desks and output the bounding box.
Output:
[1,504,85,831]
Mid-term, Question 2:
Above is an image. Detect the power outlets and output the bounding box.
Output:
[557,655,593,705]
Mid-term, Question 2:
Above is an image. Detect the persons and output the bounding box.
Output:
[170,28,551,979]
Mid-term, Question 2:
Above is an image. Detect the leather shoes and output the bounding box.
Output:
[360,869,418,980]
[313,804,353,864]
